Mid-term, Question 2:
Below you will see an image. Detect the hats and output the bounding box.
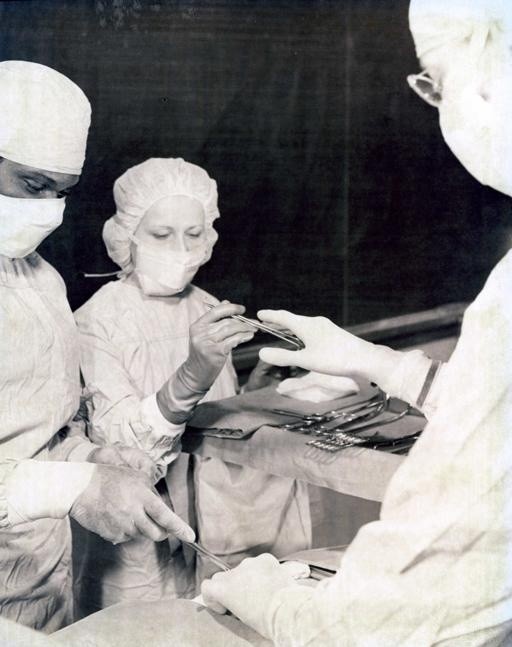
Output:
[0,60,94,177]
[102,157,219,275]
[409,1,509,59]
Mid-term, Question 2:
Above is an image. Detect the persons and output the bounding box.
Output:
[68,156,305,604]
[200,0,512,647]
[0,57,196,632]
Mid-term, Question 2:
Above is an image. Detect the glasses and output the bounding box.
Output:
[406,58,448,109]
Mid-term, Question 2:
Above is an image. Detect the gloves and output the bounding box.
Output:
[157,298,261,420]
[257,308,432,407]
[200,551,311,641]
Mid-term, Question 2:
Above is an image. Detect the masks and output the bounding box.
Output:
[0,192,68,260]
[434,99,512,201]
[114,222,207,299]
